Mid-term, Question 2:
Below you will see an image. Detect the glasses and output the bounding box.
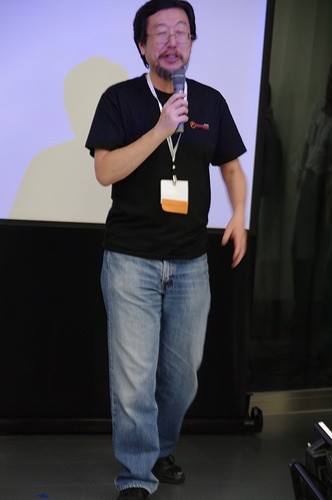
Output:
[148,30,194,44]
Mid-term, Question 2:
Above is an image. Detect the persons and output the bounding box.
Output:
[84,0,249,500]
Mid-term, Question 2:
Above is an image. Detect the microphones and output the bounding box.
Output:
[171,68,185,134]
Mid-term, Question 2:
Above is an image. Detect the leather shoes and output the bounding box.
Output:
[117,487,150,500]
[154,462,185,483]
